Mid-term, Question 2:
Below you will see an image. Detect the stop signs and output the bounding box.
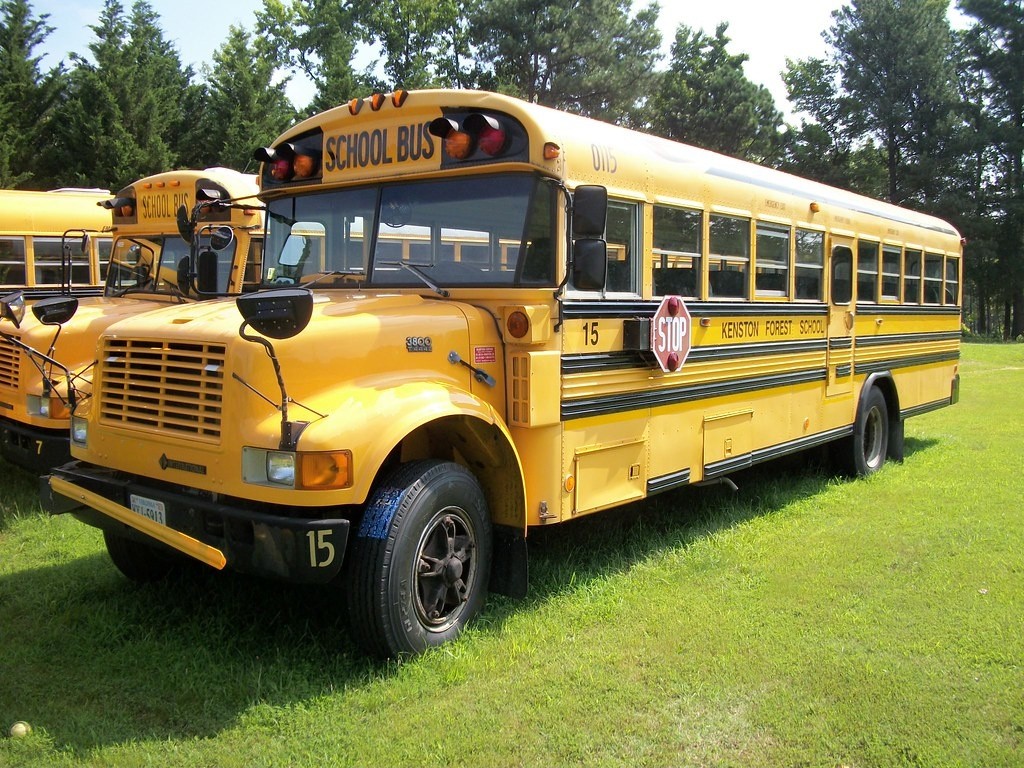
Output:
[651,295,693,373]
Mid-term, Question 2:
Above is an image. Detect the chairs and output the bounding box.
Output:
[607,259,955,302]
[375,259,516,281]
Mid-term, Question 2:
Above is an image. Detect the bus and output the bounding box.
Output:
[0,184,140,297]
[0,169,768,489]
[46,90,968,667]
